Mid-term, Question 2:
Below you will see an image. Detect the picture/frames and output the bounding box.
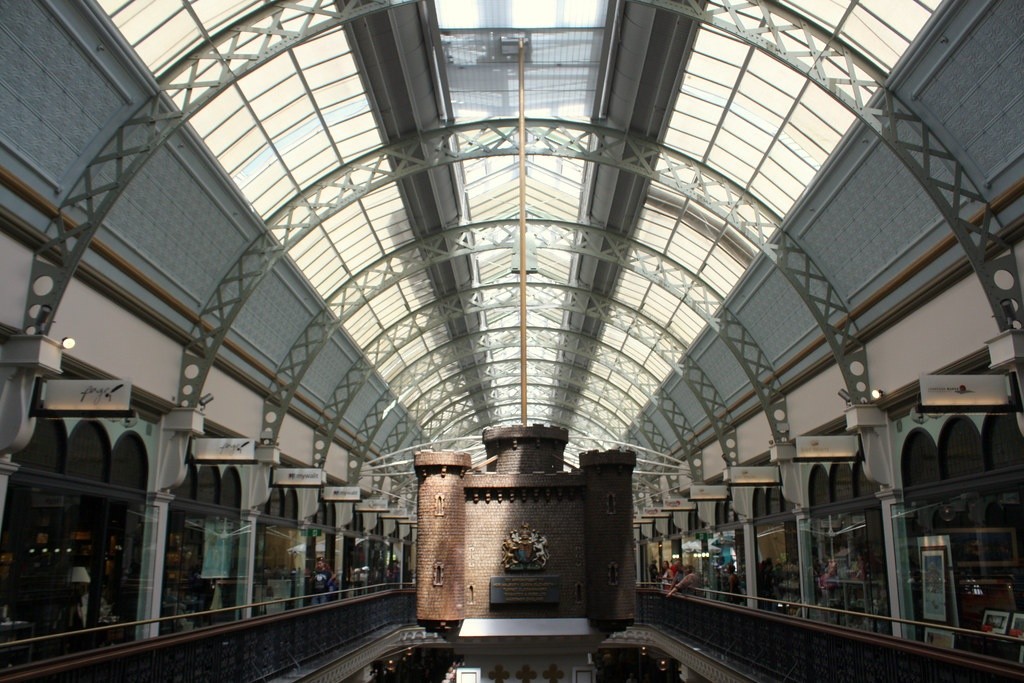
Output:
[934,526,1018,568]
[981,608,1010,635]
[919,545,951,628]
[1008,612,1024,638]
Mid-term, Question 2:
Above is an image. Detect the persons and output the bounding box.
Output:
[305,555,400,605]
[649,550,891,634]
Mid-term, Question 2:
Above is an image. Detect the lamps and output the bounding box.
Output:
[200,393,214,411]
[62,336,75,349]
[871,389,883,398]
[722,453,731,469]
[838,389,853,406]
